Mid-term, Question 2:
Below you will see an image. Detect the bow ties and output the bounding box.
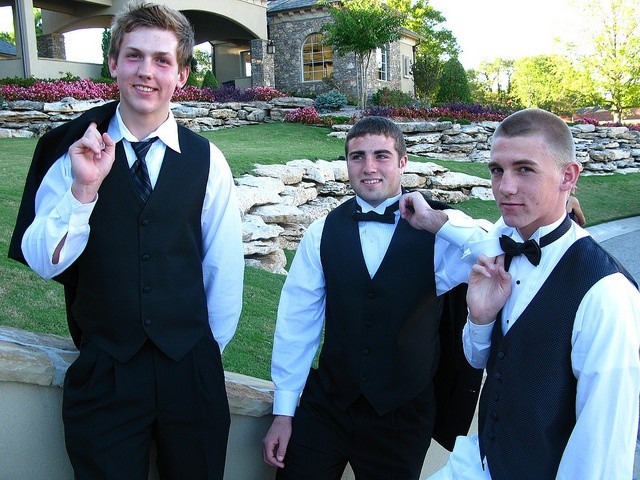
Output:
[498,214,571,273]
[355,201,401,225]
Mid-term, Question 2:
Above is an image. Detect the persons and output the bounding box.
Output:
[262,118,496,480]
[429,107,640,480]
[566,186,585,227]
[21,1,245,480]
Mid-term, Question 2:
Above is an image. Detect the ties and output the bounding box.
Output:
[127,137,157,211]
